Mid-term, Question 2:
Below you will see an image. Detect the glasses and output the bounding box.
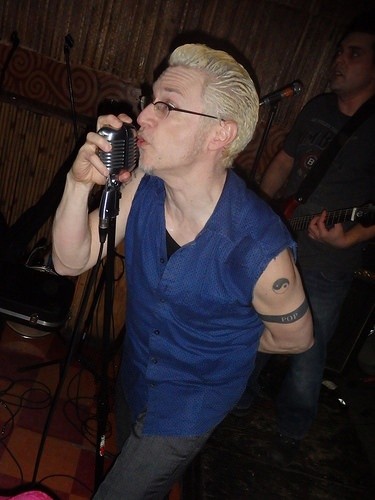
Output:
[139,94,225,122]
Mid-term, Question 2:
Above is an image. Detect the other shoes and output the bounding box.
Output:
[231,381,261,417]
[267,429,302,467]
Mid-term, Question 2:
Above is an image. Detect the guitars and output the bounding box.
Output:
[267,194,375,261]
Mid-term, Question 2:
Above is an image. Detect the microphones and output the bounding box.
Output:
[260,82,304,105]
[98,125,138,176]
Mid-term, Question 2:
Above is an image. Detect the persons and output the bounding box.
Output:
[230,14,375,443]
[51,44,316,500]
